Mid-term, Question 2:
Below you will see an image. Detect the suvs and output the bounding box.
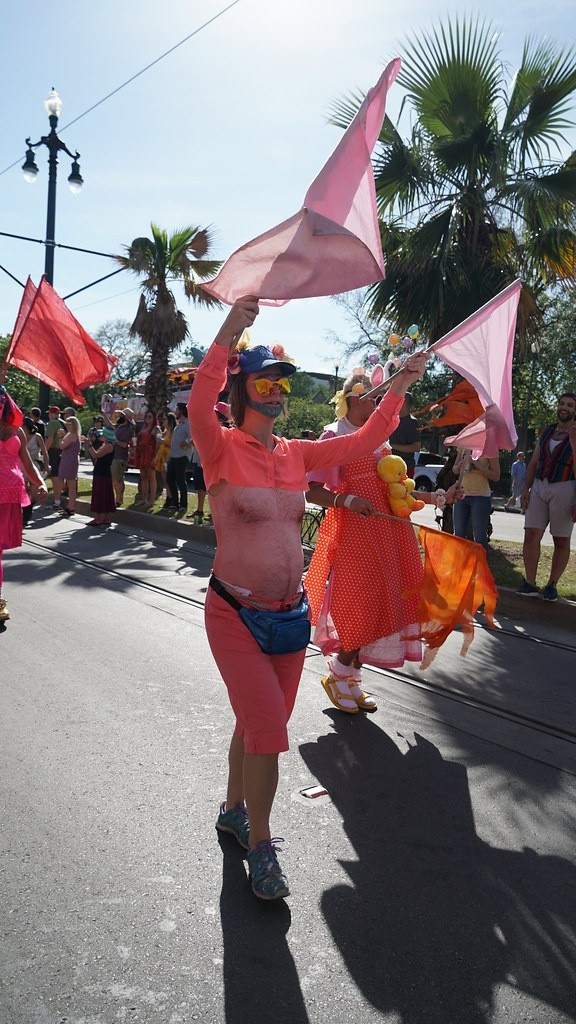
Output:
[414,451,447,493]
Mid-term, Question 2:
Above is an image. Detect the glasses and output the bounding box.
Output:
[351,394,383,407]
[0,398,7,403]
[245,377,295,395]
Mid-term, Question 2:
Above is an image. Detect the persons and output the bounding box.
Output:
[515,393,576,601]
[436,423,500,555]
[187,297,431,898]
[304,376,465,712]
[390,395,421,479]
[504,453,526,514]
[20,406,81,527]
[80,408,136,526]
[134,402,234,521]
[0,362,48,620]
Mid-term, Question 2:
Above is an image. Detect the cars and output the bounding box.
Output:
[20,87,83,423]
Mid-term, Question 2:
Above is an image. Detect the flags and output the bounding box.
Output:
[6,278,119,405]
[197,57,399,308]
[430,280,521,460]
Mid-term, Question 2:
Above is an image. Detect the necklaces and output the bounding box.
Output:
[556,425,573,436]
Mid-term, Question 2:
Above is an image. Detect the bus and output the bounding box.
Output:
[100,377,193,469]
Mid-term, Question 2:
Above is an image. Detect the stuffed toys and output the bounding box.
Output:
[378,456,425,517]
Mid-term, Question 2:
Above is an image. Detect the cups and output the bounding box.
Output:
[156,434,163,440]
[132,437,138,446]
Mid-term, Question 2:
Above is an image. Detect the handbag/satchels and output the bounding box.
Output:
[210,574,311,654]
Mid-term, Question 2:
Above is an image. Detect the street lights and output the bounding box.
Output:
[333,358,341,409]
[521,339,540,454]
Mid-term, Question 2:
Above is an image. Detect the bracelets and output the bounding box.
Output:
[435,489,446,509]
[88,445,92,447]
[344,495,358,508]
[334,494,341,508]
[59,437,63,438]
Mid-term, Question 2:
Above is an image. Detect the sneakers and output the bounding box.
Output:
[215,801,250,849]
[246,838,291,899]
[542,581,558,601]
[512,578,540,596]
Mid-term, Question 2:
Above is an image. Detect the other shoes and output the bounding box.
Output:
[45,502,75,516]
[504,504,508,513]
[100,518,111,524]
[86,519,101,525]
[113,499,213,521]
[0,597,10,618]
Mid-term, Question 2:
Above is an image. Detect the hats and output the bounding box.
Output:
[214,401,233,419]
[45,406,60,414]
[227,342,296,377]
[59,406,75,415]
[92,415,103,423]
[96,426,118,442]
[114,407,137,424]
[341,366,373,393]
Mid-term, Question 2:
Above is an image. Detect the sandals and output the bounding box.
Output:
[320,676,359,712]
[345,679,377,709]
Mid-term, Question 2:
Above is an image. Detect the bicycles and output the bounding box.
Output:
[302,507,329,573]
